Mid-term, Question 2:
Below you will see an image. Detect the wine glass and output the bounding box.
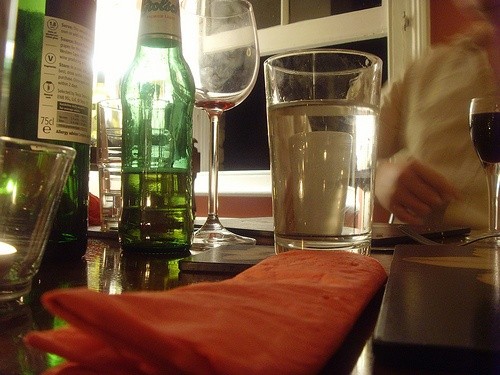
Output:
[169,0,261,250]
[465,98,500,247]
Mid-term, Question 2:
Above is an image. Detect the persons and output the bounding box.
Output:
[371,0,500,230]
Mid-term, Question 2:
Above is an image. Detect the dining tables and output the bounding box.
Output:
[0,214,500,375]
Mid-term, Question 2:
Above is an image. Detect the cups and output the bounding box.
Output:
[97,98,123,238]
[0,135,78,299]
[263,48,384,258]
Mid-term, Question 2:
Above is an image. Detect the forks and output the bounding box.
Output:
[397,225,500,247]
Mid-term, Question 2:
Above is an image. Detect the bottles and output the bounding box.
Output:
[120,0,194,256]
[0,0,97,255]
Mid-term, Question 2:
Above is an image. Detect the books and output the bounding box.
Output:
[177,243,278,274]
